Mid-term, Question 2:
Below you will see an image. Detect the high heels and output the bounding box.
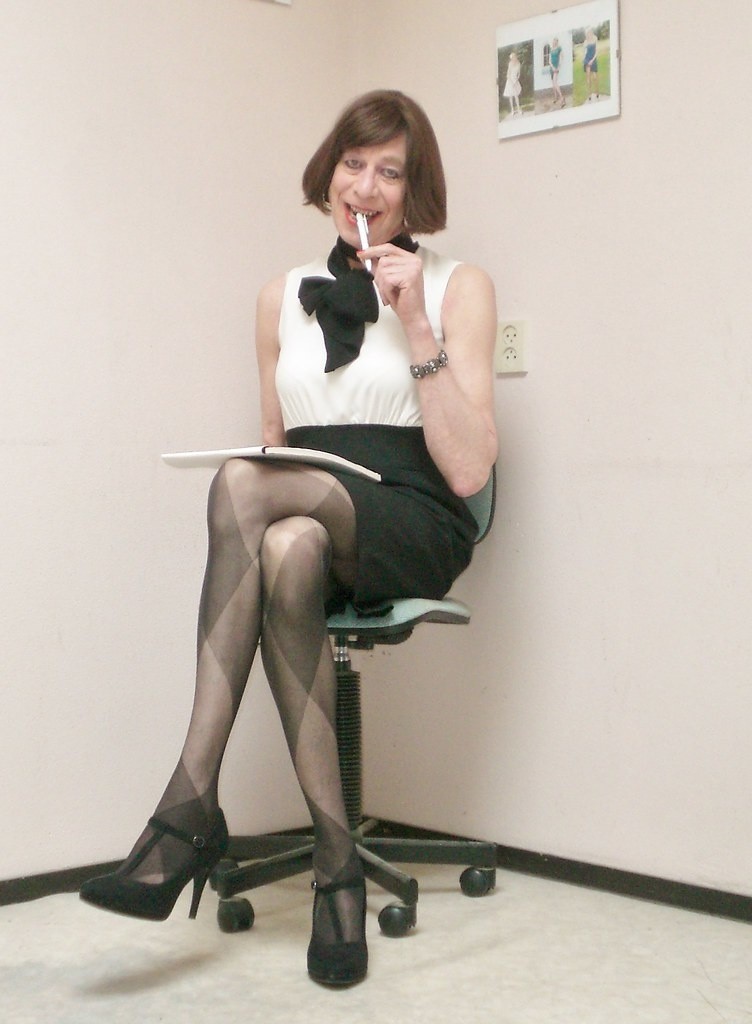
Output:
[79,807,229,922]
[307,878,369,990]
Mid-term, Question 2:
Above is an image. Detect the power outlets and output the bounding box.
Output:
[494,320,529,374]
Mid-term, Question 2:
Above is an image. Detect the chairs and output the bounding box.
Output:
[209,464,499,935]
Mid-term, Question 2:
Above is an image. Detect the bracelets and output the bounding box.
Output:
[409,350,449,379]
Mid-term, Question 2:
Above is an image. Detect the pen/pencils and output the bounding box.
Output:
[355,212,373,272]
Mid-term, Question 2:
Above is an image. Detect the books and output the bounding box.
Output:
[161,446,382,483]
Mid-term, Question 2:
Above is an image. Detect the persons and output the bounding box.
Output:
[549,36,566,108]
[583,28,600,102]
[80,90,496,988]
[503,52,524,115]
